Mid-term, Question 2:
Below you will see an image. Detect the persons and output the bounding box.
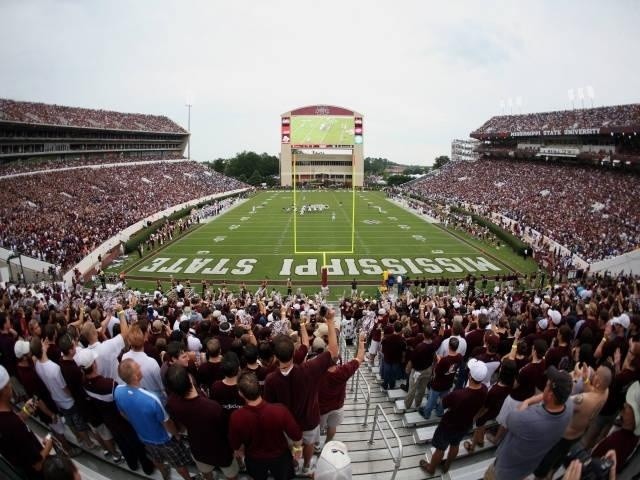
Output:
[1,275,365,478]
[312,150,325,155]
[281,202,329,217]
[384,96,640,273]
[362,271,639,479]
[1,98,259,289]
[259,182,384,191]
[332,209,336,223]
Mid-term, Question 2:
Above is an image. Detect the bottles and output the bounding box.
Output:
[195,351,201,363]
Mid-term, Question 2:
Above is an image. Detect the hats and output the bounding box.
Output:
[313,339,326,352]
[451,296,462,309]
[76,348,98,368]
[14,340,30,358]
[626,380,640,431]
[612,314,630,328]
[314,325,329,337]
[547,366,573,402]
[547,308,562,325]
[313,441,353,479]
[468,358,487,381]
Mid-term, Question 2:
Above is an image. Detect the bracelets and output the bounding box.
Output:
[117,310,125,315]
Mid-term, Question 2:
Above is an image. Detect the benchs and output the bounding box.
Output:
[368,354,567,479]
[21,406,197,480]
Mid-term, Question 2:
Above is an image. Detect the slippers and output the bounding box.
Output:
[420,459,435,475]
[464,440,474,454]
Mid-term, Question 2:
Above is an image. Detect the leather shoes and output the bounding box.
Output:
[418,407,429,419]
[400,383,409,392]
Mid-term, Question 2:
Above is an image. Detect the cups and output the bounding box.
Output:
[359,327,368,343]
